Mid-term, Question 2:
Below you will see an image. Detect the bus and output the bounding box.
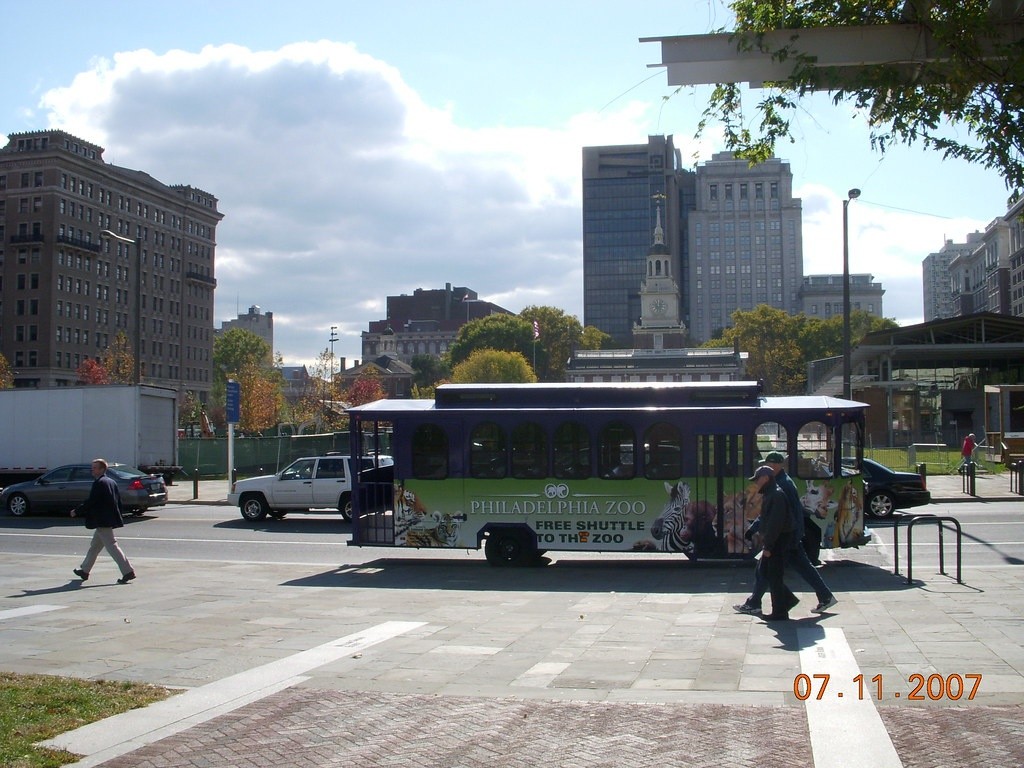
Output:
[346,376,871,568]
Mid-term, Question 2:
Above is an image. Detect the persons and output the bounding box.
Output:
[732,453,838,621]
[71,459,137,584]
[957,433,975,476]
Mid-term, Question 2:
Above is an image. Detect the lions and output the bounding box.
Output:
[711,493,757,553]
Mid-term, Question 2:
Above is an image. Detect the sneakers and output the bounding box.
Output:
[811,596,838,613]
[733,604,761,614]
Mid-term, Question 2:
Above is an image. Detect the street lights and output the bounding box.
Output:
[840,187,863,405]
[101,230,142,384]
[328,327,339,426]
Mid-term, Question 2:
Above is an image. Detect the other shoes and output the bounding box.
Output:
[760,614,791,622]
[958,468,962,474]
[74,569,89,580]
[117,572,136,583]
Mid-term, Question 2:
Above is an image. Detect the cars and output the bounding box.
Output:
[843,457,933,519]
[0,462,169,518]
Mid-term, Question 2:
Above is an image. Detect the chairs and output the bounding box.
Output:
[414,453,584,478]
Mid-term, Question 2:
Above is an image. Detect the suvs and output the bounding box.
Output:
[227,454,394,525]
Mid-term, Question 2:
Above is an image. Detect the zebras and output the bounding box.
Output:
[650,482,690,552]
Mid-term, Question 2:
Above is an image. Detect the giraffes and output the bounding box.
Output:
[800,480,837,519]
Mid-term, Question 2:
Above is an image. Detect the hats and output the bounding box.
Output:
[748,465,774,481]
[758,452,784,464]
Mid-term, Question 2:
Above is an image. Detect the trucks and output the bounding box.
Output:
[0,383,184,489]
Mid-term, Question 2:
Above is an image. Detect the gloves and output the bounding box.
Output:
[745,530,754,541]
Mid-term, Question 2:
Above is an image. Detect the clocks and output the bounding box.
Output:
[651,299,665,312]
